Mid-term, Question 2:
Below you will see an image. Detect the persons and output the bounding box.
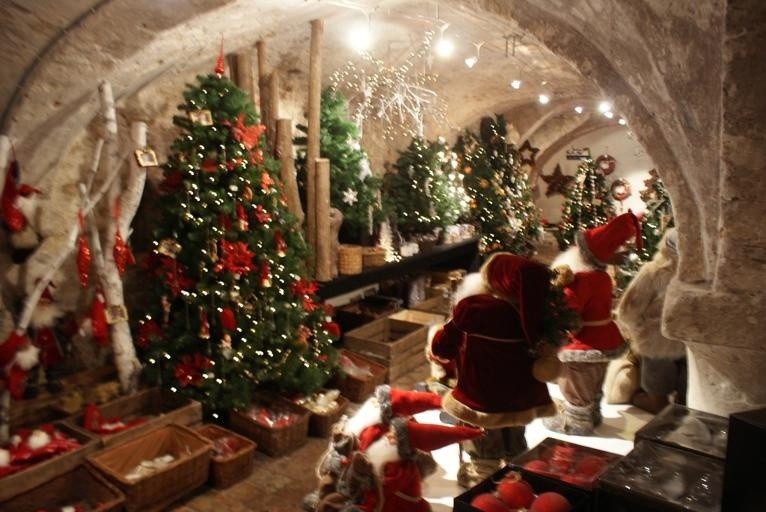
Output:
[613,225,688,415]
[540,209,646,438]
[423,251,585,488]
[315,382,488,511]
